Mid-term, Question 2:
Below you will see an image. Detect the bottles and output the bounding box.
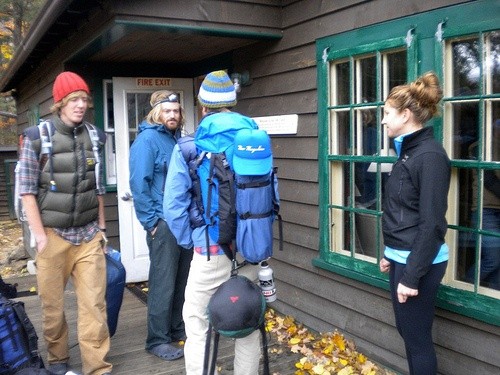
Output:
[257,262,277,302]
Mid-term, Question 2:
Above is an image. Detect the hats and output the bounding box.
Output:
[52,71,89,103]
[232,127,273,175]
[197,70,237,109]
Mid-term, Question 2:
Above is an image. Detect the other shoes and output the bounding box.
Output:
[48,363,68,375]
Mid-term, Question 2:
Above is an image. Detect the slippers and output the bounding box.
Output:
[147,343,184,361]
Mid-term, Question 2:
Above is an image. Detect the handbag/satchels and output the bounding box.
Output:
[103,253,126,336]
[206,276,267,338]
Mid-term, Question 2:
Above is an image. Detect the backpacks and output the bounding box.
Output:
[0,290,44,375]
[187,113,281,264]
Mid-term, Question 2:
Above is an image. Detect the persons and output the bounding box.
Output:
[129,90,194,360]
[162,70,280,375]
[467,119,500,290]
[379,71,450,374]
[17,71,113,375]
[349,89,391,212]
